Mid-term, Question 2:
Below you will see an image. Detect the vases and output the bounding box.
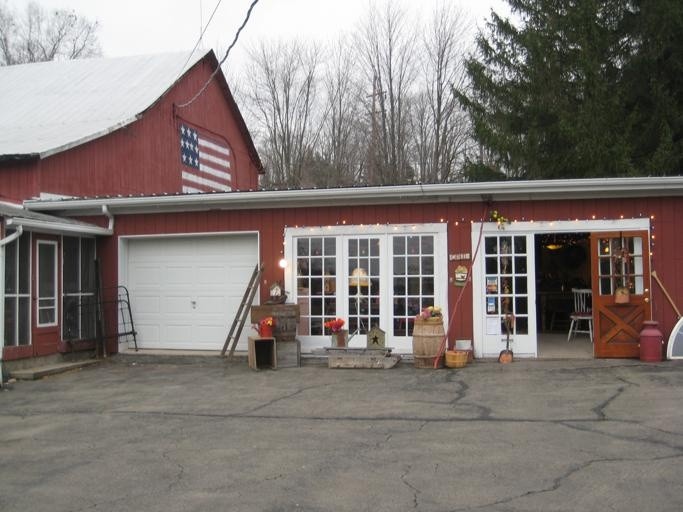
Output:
[330,330,348,348]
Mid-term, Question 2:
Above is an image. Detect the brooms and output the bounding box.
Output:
[615,232,630,303]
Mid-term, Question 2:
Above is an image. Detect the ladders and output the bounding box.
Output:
[220,264,265,359]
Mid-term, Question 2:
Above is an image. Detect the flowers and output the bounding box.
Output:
[414,306,442,320]
[324,318,344,332]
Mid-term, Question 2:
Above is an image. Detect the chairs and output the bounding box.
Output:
[567,287,593,344]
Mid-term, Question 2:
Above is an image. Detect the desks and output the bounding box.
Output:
[537,288,562,330]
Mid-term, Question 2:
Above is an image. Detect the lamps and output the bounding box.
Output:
[543,233,565,250]
[348,268,372,333]
